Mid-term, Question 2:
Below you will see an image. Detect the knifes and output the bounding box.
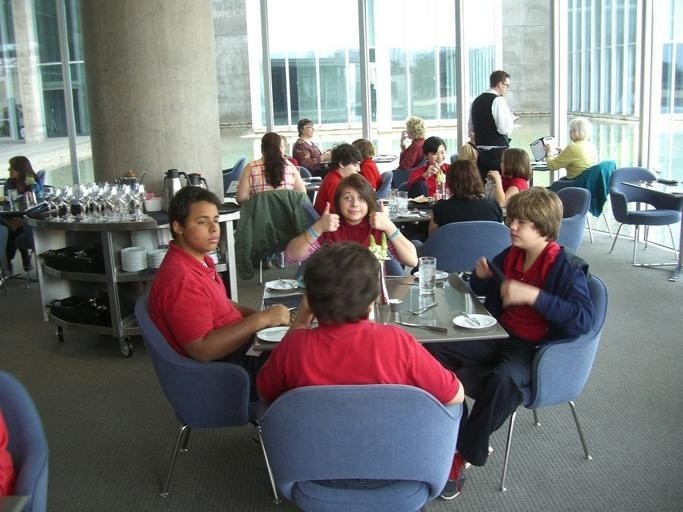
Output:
[458,310,482,324]
[279,277,294,289]
[412,302,437,316]
[395,318,449,332]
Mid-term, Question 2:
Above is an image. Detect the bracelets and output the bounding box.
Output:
[303,231,311,244]
[388,229,401,240]
[307,227,320,239]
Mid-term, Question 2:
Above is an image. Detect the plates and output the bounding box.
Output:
[147,249,167,270]
[265,277,297,290]
[208,251,219,264]
[120,246,148,273]
[130,230,157,249]
[255,327,288,343]
[451,311,499,330]
[413,270,448,281]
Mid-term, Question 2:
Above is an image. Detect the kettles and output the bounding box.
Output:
[187,172,208,189]
[163,168,190,210]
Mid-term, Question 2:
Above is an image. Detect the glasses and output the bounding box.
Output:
[502,83,510,88]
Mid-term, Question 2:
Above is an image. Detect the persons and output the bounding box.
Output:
[398,117,427,170]
[408,136,451,196]
[279,135,299,167]
[468,71,516,184]
[312,144,362,216]
[237,132,307,269]
[353,139,382,190]
[3,156,45,271]
[258,242,464,489]
[542,118,597,180]
[424,187,596,501]
[287,174,418,267]
[488,148,533,207]
[150,187,291,401]
[428,160,503,238]
[459,115,485,189]
[292,119,332,179]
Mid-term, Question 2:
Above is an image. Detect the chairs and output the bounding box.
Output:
[0,366,50,511]
[223,157,246,197]
[294,165,312,183]
[254,386,464,512]
[294,244,407,279]
[610,168,683,269]
[374,171,394,199]
[503,186,591,255]
[498,271,608,490]
[548,161,616,244]
[393,155,431,187]
[415,221,514,292]
[136,290,255,498]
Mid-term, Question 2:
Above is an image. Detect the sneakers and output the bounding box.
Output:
[439,476,466,501]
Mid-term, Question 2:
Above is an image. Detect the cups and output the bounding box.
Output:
[419,257,439,293]
[395,190,408,212]
[7,189,19,212]
[435,178,445,200]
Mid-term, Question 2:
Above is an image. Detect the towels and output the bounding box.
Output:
[263,293,305,311]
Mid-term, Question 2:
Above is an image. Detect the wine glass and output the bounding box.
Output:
[39,183,147,223]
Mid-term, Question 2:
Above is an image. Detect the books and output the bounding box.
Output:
[531,136,560,161]
